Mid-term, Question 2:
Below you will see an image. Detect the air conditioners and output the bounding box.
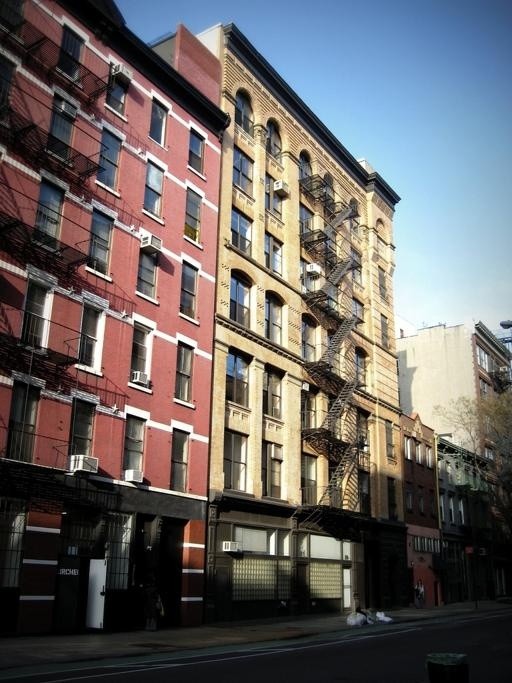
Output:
[112,63,133,82]
[306,263,321,275]
[124,470,144,483]
[223,541,239,552]
[70,454,98,474]
[274,179,289,196]
[130,370,148,385]
[140,234,162,254]
